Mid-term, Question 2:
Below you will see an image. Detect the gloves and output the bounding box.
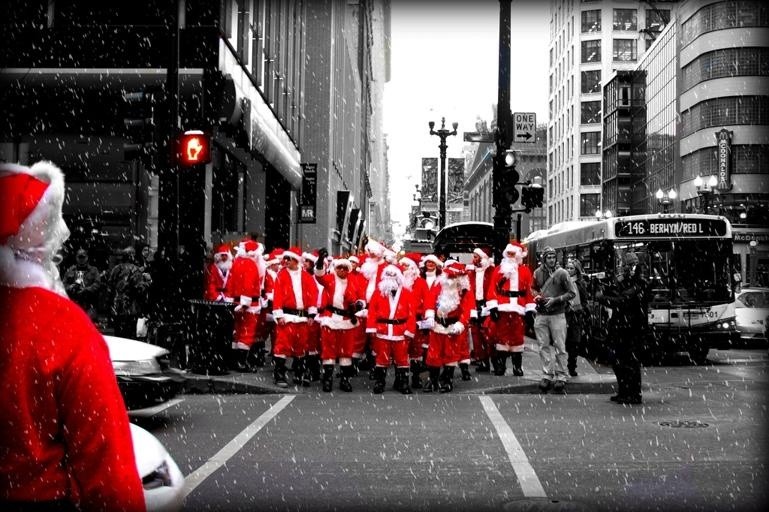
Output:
[522,309,536,326]
[315,247,329,271]
[489,307,503,320]
[354,299,368,311]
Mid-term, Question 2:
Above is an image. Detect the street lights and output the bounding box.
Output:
[656,186,678,212]
[427,115,460,233]
[592,207,613,225]
[746,238,759,288]
[690,173,719,214]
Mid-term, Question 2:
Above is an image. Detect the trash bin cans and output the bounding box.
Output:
[186,300,236,376]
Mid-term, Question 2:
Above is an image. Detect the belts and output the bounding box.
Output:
[473,296,488,309]
[377,318,409,324]
[282,305,310,319]
[232,296,259,303]
[259,289,267,296]
[323,303,354,320]
[414,314,424,321]
[215,286,227,293]
[498,287,527,298]
[434,315,458,326]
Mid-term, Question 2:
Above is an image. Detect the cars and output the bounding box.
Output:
[99,332,188,419]
[730,284,769,348]
[124,421,187,512]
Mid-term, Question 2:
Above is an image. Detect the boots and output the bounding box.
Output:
[474,349,492,373]
[273,351,457,393]
[457,361,474,382]
[230,340,270,375]
[510,352,525,377]
[493,349,508,377]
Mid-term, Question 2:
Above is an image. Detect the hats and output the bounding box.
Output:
[333,254,352,272]
[363,238,397,263]
[425,254,441,265]
[473,246,493,263]
[503,239,527,260]
[442,259,466,277]
[384,263,407,277]
[539,245,558,261]
[213,240,319,266]
[398,251,422,267]
[567,257,582,274]
[0,156,69,258]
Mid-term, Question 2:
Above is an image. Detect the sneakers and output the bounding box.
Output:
[552,379,566,392]
[539,376,553,390]
[566,357,579,377]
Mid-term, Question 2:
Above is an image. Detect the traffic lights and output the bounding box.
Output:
[176,128,209,167]
[498,148,522,207]
[117,79,163,173]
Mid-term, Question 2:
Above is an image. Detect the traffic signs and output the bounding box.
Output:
[512,110,538,144]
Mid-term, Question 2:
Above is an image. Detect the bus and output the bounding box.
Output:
[520,210,740,368]
[429,220,497,268]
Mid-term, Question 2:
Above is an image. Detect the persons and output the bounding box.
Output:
[62,237,589,394]
[0,158,147,511]
[592,251,649,404]
